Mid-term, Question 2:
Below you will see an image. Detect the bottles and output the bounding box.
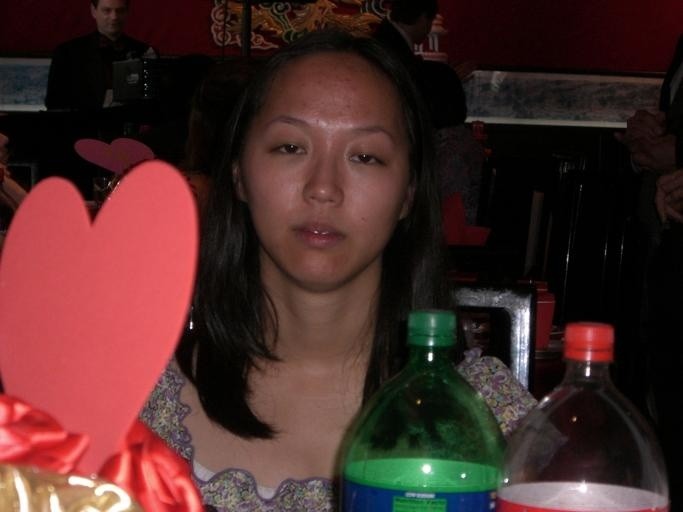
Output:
[334,308,509,512]
[496,322,668,512]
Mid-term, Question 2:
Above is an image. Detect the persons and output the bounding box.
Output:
[137,30,539,511]
[45,0,159,201]
[622,34,683,385]
